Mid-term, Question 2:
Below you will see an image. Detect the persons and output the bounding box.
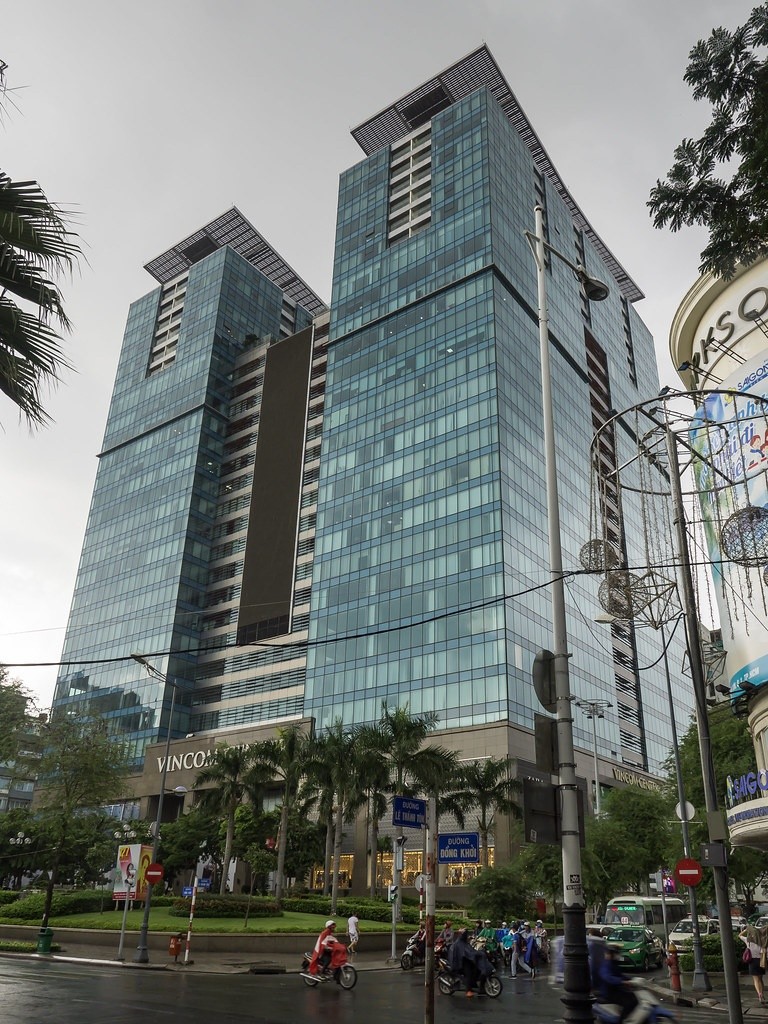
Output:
[317,921,342,979]
[164,877,173,896]
[410,920,549,999]
[738,926,768,1007]
[346,910,360,953]
[595,947,642,1024]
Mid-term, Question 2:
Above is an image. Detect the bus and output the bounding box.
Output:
[605,894,688,948]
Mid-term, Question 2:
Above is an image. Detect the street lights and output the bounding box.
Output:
[110,823,137,961]
[9,831,32,891]
[574,699,613,822]
[525,228,613,1024]
[129,652,182,963]
[137,785,188,963]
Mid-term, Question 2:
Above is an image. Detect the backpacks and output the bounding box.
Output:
[516,933,527,953]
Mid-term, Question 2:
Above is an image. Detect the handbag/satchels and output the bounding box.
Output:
[742,941,751,963]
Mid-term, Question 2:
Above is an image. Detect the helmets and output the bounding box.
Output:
[502,922,507,926]
[446,921,451,928]
[476,920,482,927]
[524,922,530,926]
[484,919,491,923]
[604,942,620,954]
[326,920,336,928]
[418,921,426,929]
[537,920,542,924]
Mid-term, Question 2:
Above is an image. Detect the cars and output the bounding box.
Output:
[551,924,665,974]
[688,913,768,935]
[665,919,720,960]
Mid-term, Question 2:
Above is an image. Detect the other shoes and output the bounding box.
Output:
[508,976,517,980]
[466,991,473,998]
[346,947,351,954]
[530,968,535,979]
[352,951,355,952]
[319,972,325,978]
[758,993,767,1004]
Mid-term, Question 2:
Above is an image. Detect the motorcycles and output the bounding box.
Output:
[400,936,426,970]
[299,940,358,989]
[433,954,504,999]
[433,937,551,973]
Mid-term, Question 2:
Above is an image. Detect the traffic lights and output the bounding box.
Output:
[388,885,399,902]
[649,873,663,892]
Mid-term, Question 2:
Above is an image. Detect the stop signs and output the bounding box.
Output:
[675,859,703,886]
[145,863,164,884]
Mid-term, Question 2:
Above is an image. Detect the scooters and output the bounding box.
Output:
[593,977,680,1024]
